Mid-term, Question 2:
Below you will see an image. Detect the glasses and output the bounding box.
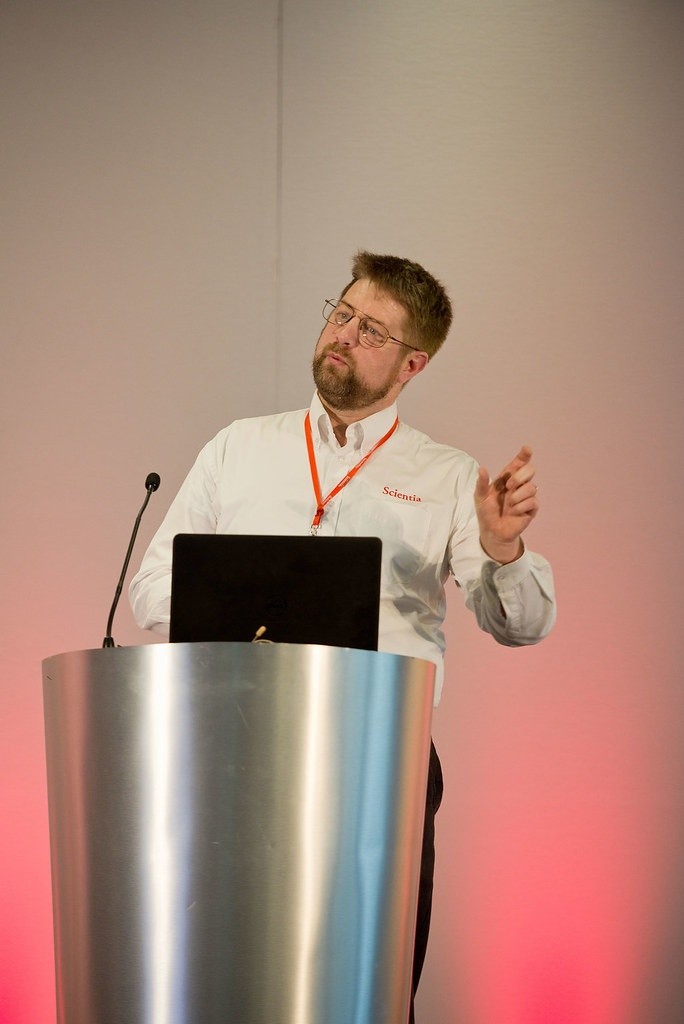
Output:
[321,297,418,351]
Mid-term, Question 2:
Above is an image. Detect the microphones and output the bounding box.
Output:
[104,472,160,648]
[253,626,265,642]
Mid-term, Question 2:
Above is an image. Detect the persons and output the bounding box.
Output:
[128,250,556,1024]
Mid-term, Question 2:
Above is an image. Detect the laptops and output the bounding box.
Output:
[169,534,383,653]
